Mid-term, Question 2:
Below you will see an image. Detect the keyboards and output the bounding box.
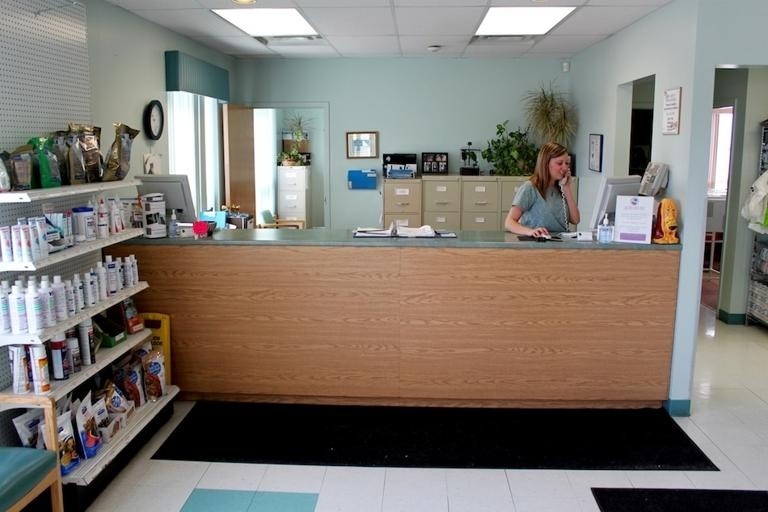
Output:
[559,231,581,238]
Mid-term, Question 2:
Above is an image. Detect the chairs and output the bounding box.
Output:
[0,394,64,512]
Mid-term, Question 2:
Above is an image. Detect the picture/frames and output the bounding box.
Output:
[345,131,379,160]
[421,152,449,175]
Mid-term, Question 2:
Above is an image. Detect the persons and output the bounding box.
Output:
[504,142,580,238]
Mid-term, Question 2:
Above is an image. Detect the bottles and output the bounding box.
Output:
[73,193,126,244]
[168,209,180,238]
[0,255,138,334]
[8,318,96,395]
[597,213,616,244]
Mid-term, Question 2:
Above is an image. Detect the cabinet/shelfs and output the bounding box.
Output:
[384,176,530,231]
[277,165,313,227]
[0,179,180,487]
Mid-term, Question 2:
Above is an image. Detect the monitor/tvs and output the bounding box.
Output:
[589,175,641,237]
[134,175,197,224]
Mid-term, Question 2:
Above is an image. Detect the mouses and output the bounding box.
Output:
[534,233,551,239]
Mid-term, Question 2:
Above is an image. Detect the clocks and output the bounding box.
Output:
[143,101,164,141]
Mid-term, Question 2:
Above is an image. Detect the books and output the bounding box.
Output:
[352,223,437,238]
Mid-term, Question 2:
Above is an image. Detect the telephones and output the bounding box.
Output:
[557,167,571,186]
